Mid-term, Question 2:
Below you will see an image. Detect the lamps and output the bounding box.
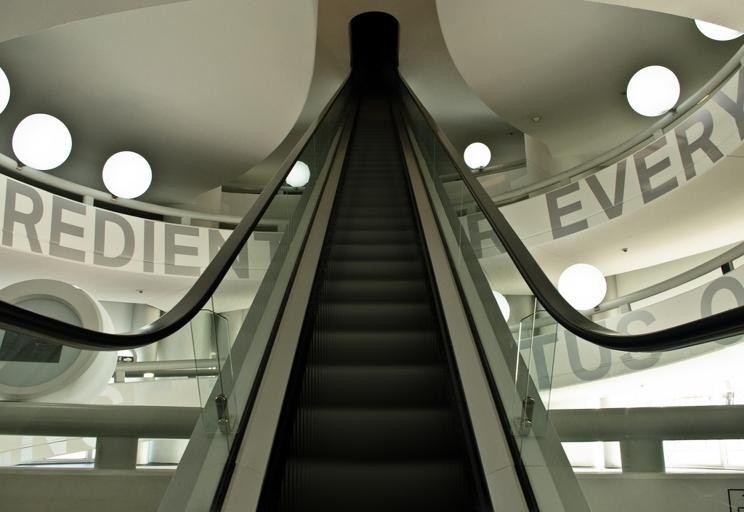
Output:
[284,158,311,190]
[694,19,744,46]
[558,264,609,311]
[625,64,680,116]
[0,66,74,172]
[463,139,493,172]
[101,147,155,202]
